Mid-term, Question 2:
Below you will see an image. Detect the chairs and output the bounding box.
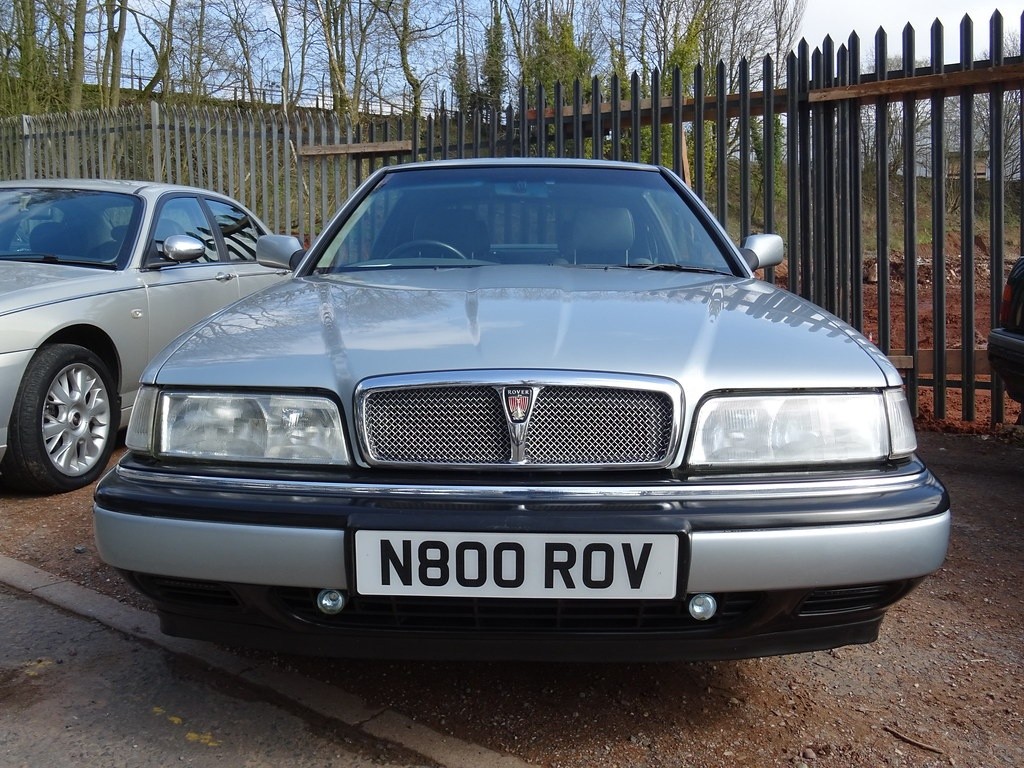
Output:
[552,206,651,268]
[412,208,479,261]
[29,220,189,268]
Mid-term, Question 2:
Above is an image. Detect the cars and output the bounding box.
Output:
[0,178,292,497]
[92,156,951,665]
